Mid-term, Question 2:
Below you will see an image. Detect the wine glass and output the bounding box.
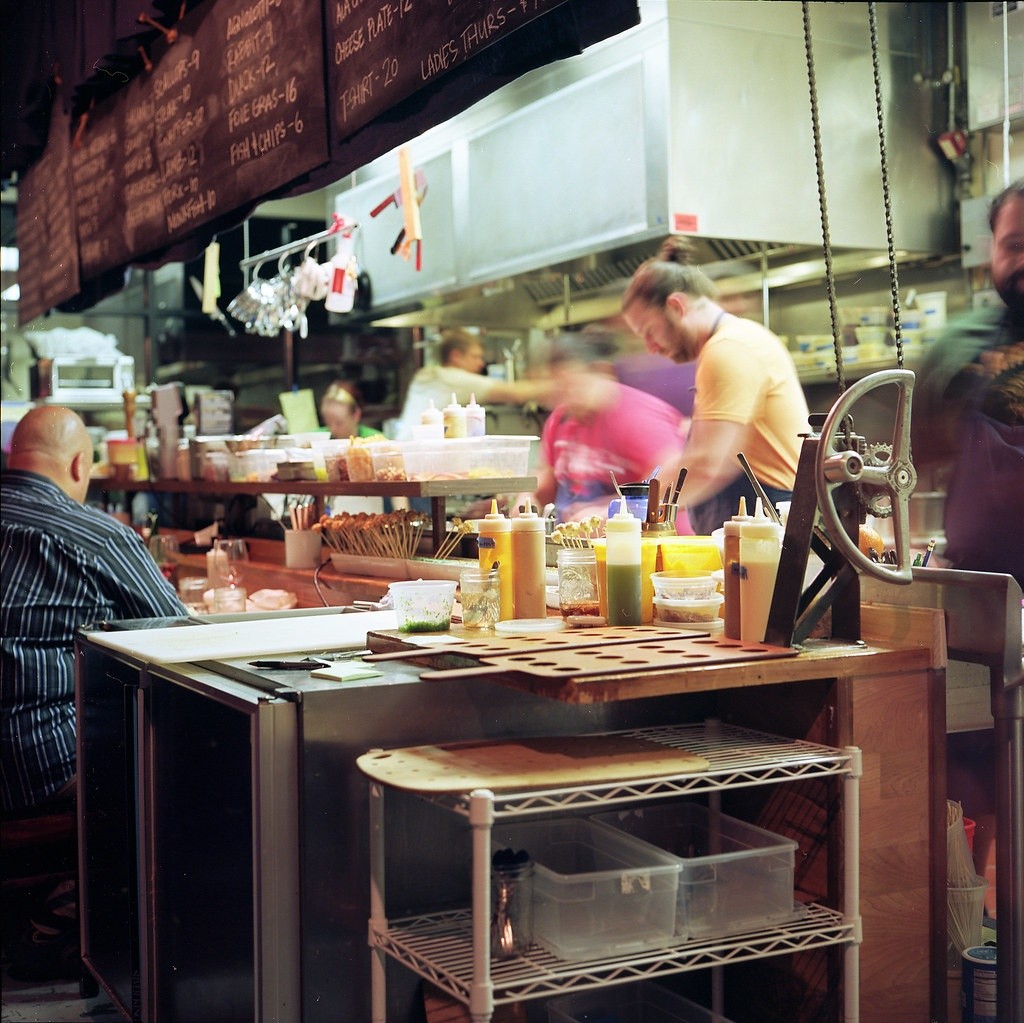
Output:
[215,539,248,613]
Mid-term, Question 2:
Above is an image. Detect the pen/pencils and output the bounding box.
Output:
[866,537,937,568]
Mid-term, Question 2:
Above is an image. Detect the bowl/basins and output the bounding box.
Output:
[388,579,458,632]
[179,577,299,615]
[651,571,724,635]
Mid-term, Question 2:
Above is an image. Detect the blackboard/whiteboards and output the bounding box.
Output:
[13,1,565,337]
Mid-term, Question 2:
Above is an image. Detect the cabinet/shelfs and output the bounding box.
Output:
[368,718,862,1023]
[88,478,538,608]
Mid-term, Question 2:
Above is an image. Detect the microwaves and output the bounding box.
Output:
[30,356,135,403]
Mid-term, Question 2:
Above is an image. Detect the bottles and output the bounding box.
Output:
[478,497,546,621]
[423,393,489,439]
[605,496,643,626]
[207,538,228,590]
[723,496,782,642]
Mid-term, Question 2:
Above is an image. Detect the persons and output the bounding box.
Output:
[891,177,1024,936]
[475,324,695,534]
[397,328,553,438]
[614,231,817,540]
[0,399,206,980]
[315,380,385,438]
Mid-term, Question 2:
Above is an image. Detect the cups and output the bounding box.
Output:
[592,536,722,624]
[284,529,323,569]
[556,548,600,622]
[459,569,500,628]
[489,860,537,959]
[148,534,179,580]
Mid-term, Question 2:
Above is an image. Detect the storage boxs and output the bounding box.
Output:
[491,801,801,962]
